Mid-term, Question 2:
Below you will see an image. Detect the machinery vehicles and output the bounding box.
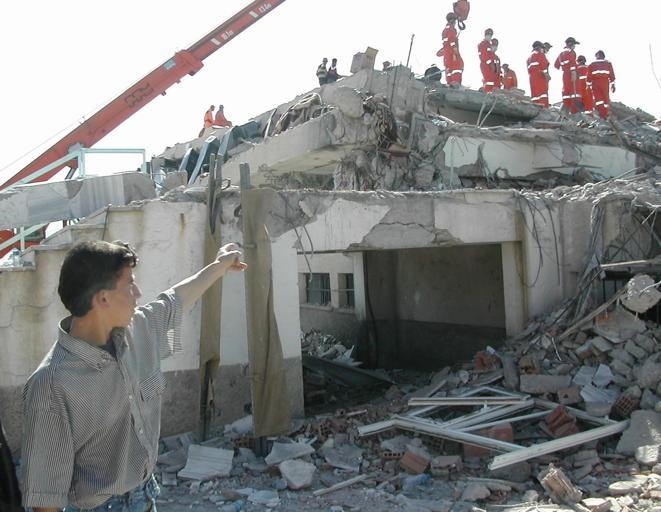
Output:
[0,0,470,257]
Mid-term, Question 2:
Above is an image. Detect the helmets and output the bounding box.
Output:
[446,12,456,21]
[489,38,498,46]
[484,28,493,35]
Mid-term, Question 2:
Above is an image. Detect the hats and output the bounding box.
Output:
[566,37,580,45]
[532,41,544,48]
[594,50,605,57]
[541,42,553,49]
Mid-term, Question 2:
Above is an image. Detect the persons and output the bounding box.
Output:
[20,239,248,512]
[425,13,616,121]
[317,57,341,87]
[204,105,232,130]
